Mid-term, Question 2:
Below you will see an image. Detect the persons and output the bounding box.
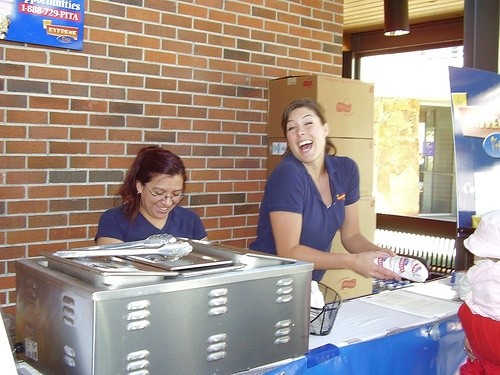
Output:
[94,146,209,248]
[455,206,500,375]
[248,97,403,282]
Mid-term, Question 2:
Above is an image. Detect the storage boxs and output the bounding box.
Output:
[265,74,376,305]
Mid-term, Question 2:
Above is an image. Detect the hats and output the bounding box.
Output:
[463,211,500,259]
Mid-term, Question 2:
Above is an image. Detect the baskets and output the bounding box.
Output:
[309,280,342,336]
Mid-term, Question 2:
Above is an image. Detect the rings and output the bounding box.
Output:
[463,346,466,350]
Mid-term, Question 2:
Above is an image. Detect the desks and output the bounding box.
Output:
[233,274,466,375]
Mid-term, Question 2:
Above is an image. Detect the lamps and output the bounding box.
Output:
[384,0,410,37]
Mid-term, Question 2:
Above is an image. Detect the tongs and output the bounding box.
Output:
[52,233,193,261]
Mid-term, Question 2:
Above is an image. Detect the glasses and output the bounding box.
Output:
[143,183,184,203]
